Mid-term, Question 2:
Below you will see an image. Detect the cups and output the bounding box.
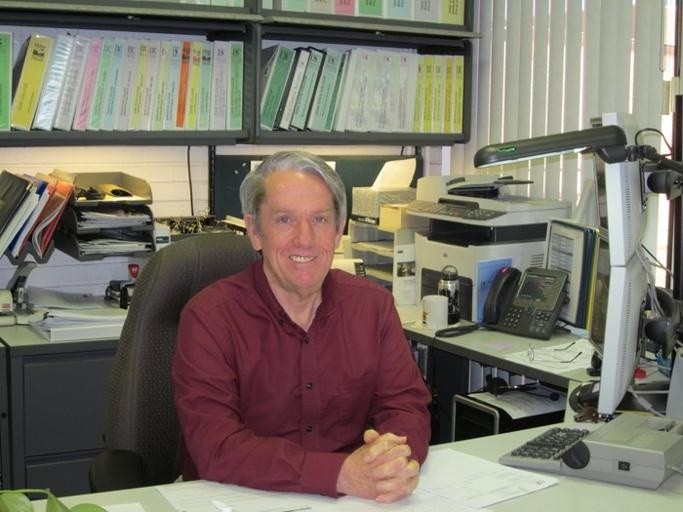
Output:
[420,295,450,329]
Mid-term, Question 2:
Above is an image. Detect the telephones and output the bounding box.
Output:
[483,266,568,340]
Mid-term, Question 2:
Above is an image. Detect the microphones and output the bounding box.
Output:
[509,389,559,402]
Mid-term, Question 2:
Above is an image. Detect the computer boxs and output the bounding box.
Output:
[450,379,570,443]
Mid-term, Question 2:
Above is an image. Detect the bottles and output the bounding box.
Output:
[437,264,461,325]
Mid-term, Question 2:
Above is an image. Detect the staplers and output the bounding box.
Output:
[0,290,15,326]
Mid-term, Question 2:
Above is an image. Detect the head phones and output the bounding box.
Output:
[486,376,538,394]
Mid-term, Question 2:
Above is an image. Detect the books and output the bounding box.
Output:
[0,170,153,344]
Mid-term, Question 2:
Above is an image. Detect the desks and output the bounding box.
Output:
[0,304,132,499]
[400,318,678,438]
[13,412,681,510]
[0,338,10,491]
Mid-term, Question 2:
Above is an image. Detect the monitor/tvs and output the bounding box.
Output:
[589,235,659,416]
[591,112,649,266]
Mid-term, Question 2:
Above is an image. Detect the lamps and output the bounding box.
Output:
[472,123,683,415]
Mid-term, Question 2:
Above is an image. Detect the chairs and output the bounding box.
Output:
[83,232,267,492]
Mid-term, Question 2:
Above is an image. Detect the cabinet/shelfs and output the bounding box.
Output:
[46,167,161,262]
[0,0,484,152]
[345,218,426,309]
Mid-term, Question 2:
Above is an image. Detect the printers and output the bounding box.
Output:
[406,176,572,327]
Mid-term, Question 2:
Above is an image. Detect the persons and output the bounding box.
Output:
[171,149,434,505]
